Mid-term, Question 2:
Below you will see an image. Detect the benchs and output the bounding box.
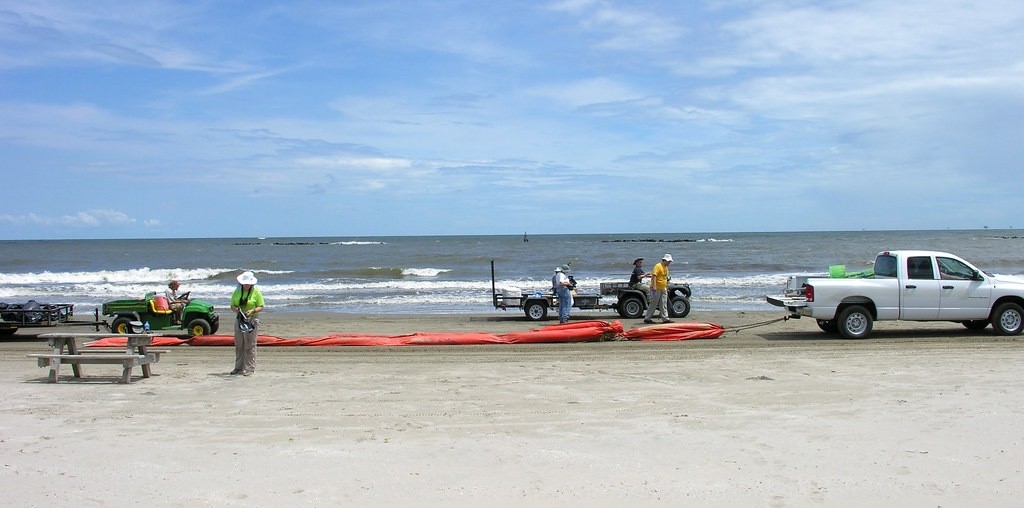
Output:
[23,353,153,384]
[55,349,166,378]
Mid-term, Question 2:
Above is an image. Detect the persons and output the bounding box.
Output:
[164,281,189,324]
[644,253,675,324]
[930,260,969,280]
[628,257,651,306]
[230,272,265,376]
[552,264,573,324]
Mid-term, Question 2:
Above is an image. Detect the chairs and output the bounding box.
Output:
[149,299,173,314]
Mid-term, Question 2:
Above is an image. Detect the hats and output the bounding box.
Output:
[633,257,644,265]
[170,280,181,287]
[237,272,257,285]
[661,253,673,262]
[554,267,561,271]
[559,264,571,273]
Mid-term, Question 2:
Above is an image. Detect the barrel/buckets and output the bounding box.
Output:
[829,265,846,279]
[155,294,169,311]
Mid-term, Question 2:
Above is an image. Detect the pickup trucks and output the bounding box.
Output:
[766,250,1024,340]
[101,290,221,339]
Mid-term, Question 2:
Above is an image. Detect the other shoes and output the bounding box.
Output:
[231,369,244,374]
[174,321,182,324]
[662,319,675,324]
[644,319,656,324]
[244,371,254,376]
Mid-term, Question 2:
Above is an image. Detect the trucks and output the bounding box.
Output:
[490,258,692,322]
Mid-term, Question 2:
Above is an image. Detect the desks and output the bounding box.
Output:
[39,332,163,383]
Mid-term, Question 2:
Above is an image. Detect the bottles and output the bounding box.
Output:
[536,291,541,298]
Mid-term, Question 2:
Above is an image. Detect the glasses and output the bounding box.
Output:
[638,261,643,264]
